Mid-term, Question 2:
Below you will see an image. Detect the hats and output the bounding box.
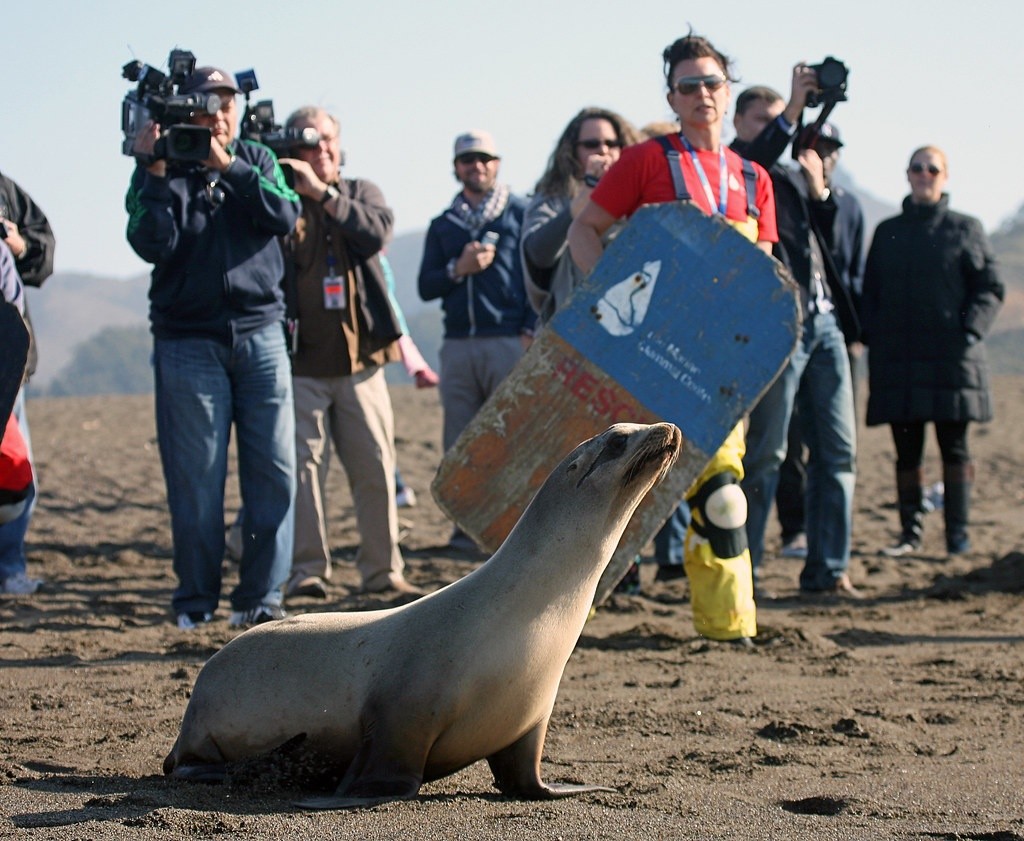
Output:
[455,129,501,161]
[182,66,243,95]
[805,122,843,147]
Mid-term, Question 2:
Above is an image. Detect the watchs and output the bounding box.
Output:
[320,185,337,205]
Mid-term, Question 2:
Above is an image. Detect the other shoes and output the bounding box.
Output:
[176,612,213,628]
[227,525,243,561]
[415,368,440,388]
[718,637,756,650]
[293,576,327,598]
[945,529,972,553]
[4,573,46,594]
[398,488,417,506]
[229,605,275,626]
[877,541,925,557]
[782,538,808,559]
[801,573,875,606]
[366,571,422,593]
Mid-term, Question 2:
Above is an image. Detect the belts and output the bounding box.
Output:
[808,300,835,313]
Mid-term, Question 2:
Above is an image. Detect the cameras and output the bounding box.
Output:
[803,57,849,107]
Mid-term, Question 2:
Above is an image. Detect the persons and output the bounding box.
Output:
[568,34,781,645]
[125,65,1007,628]
[0,171,55,596]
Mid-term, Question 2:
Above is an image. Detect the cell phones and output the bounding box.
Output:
[481,231,498,244]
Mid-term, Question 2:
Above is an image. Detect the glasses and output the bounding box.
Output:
[674,74,726,94]
[911,162,940,174]
[461,154,496,164]
[321,135,336,142]
[578,139,620,148]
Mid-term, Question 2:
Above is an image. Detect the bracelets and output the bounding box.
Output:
[224,154,238,173]
[583,175,599,188]
[821,186,831,201]
